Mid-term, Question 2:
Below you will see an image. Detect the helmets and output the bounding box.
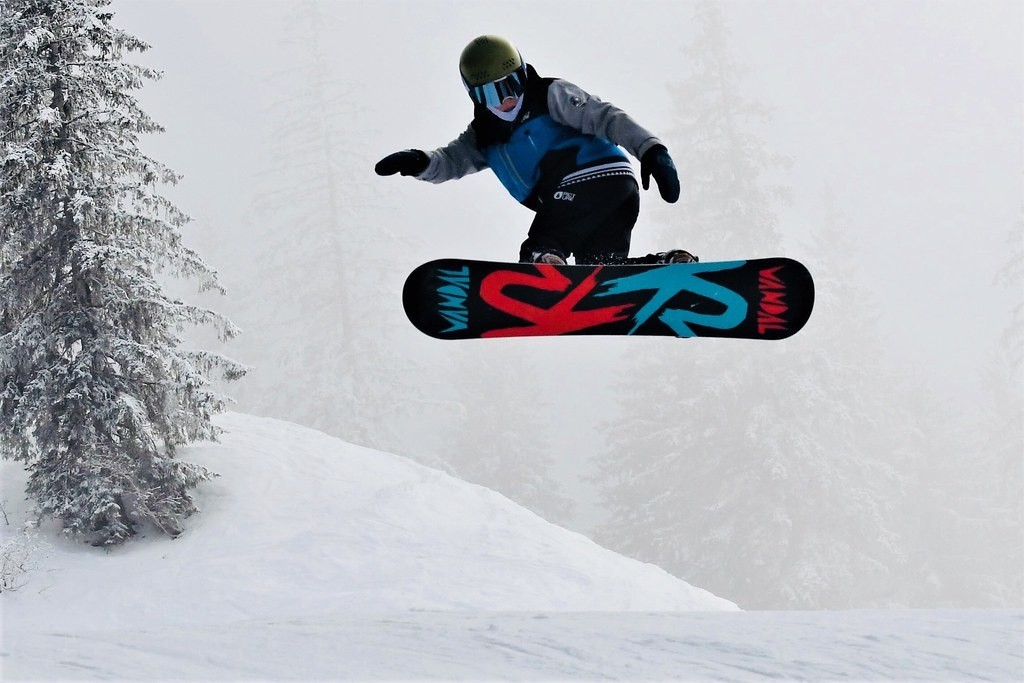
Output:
[462,35,524,93]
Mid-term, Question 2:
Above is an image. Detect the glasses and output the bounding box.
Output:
[470,63,528,107]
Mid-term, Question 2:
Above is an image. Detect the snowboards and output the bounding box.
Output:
[403,256,817,342]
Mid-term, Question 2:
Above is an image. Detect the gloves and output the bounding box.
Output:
[641,146,681,204]
[374,149,430,176]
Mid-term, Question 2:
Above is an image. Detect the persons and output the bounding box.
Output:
[376,36,700,265]
